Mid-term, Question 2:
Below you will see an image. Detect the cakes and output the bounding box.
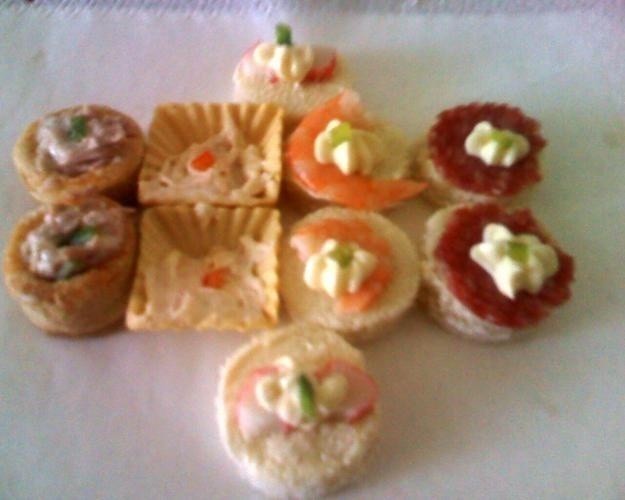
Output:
[12,103,144,206]
[212,320,383,500]
[419,202,577,343]
[277,207,420,347]
[137,101,283,206]
[126,204,281,330]
[3,192,138,337]
[413,103,546,207]
[283,86,429,214]
[231,21,361,131]
